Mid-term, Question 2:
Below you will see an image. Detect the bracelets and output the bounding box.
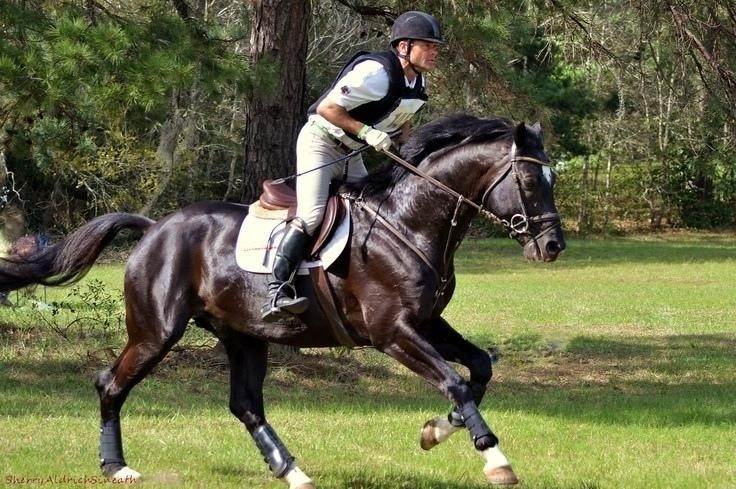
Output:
[357,124,369,139]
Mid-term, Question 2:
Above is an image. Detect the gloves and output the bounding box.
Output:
[361,125,392,152]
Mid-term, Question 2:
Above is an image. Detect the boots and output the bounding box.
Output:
[261,226,311,323]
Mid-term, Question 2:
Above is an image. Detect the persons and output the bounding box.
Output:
[260,9,445,324]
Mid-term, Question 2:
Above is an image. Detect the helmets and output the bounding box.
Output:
[389,11,445,46]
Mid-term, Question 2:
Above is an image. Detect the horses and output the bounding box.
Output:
[0,111,567,489]
